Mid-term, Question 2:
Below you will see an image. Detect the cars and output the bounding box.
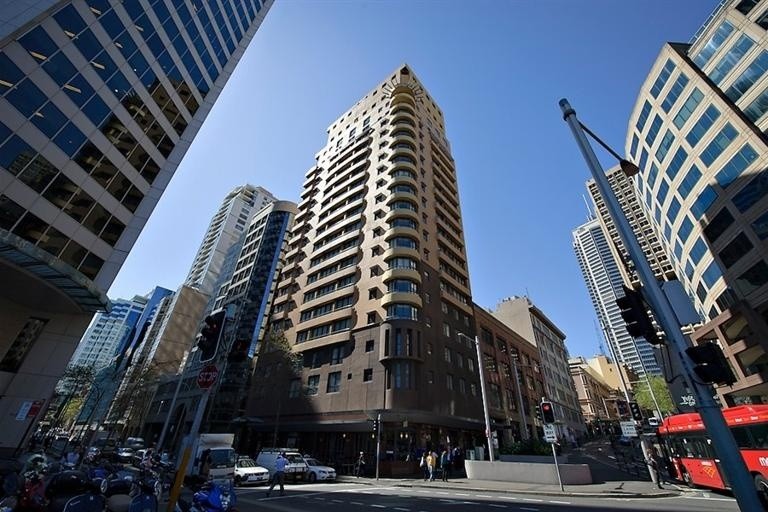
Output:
[618,418,667,468]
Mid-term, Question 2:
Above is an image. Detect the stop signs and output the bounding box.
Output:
[196,365,219,390]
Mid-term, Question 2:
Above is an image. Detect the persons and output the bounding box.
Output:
[60,446,82,470]
[198,448,213,477]
[28,433,54,452]
[356,450,368,478]
[419,449,450,482]
[266,453,289,497]
[646,448,665,489]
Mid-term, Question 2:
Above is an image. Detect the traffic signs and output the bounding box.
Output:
[542,424,557,441]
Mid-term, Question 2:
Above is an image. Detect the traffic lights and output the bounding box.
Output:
[540,401,556,424]
[615,285,659,346]
[373,419,377,435]
[616,399,629,415]
[197,309,227,363]
[535,403,544,424]
[684,342,734,387]
[627,401,643,422]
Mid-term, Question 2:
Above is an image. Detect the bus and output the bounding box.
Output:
[655,403,768,508]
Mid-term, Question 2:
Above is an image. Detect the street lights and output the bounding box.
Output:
[513,358,543,440]
[559,98,765,512]
[457,332,495,462]
